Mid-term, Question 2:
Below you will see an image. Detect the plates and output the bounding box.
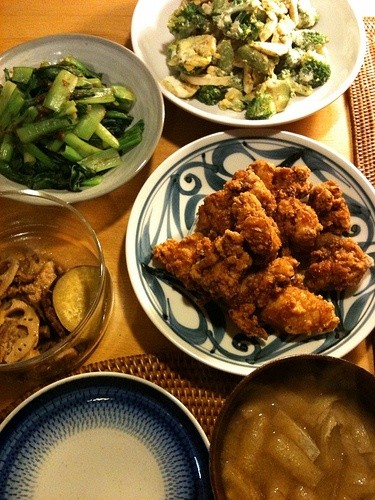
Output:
[124,129,375,376]
[1,33,165,204]
[131,0,368,127]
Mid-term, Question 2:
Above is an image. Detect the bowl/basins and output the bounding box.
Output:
[209,355,375,500]
[0,189,114,374]
[0,372,211,499]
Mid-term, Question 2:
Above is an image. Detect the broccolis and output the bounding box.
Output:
[167,0,332,120]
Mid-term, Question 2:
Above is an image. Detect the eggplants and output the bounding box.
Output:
[47,264,109,341]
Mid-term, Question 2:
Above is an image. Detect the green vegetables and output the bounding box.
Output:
[0,55,146,192]
